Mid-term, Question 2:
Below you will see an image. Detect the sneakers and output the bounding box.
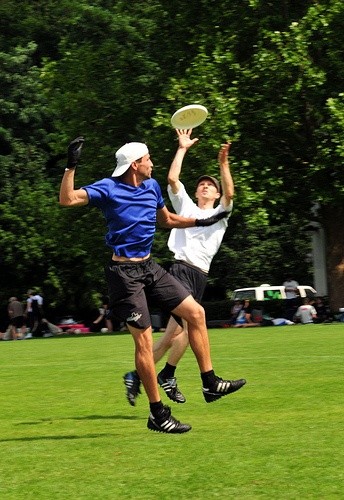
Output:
[147,405,192,433]
[123,370,142,406]
[157,375,186,403]
[202,378,246,403]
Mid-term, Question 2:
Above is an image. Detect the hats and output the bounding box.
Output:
[111,142,149,177]
[197,175,220,193]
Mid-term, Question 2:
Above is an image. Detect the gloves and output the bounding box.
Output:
[67,136,86,168]
[195,211,230,226]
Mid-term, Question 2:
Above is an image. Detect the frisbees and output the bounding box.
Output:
[171,105,208,129]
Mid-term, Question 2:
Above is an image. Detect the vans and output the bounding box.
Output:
[233,283,317,301]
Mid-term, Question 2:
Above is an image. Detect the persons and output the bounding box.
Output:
[231,296,340,327]
[123,128,234,406]
[59,136,246,434]
[7,288,50,341]
[100,296,113,333]
[283,275,300,299]
